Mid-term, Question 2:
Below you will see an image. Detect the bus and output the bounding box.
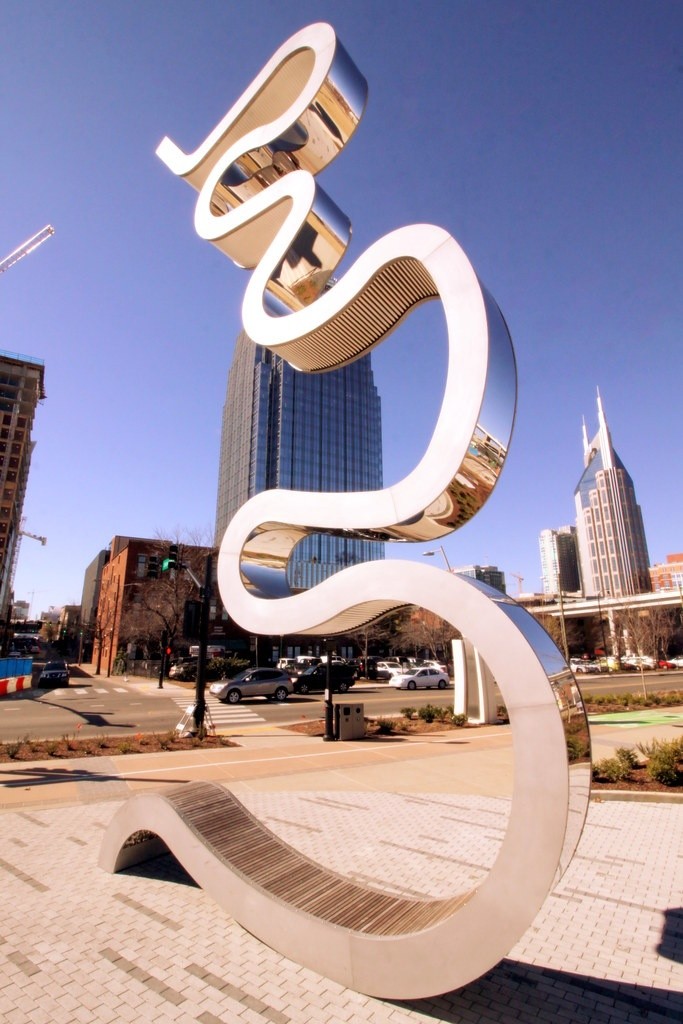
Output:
[11,632,43,654]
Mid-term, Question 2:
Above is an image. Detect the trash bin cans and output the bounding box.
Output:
[335,700,365,741]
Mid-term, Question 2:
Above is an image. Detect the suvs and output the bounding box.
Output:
[37,660,70,688]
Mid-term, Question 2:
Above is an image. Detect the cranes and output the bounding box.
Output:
[4,515,48,625]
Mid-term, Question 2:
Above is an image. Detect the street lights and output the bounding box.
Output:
[597,587,612,675]
[422,545,452,572]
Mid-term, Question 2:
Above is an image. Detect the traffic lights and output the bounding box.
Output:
[161,630,168,647]
[168,544,178,569]
[166,637,174,655]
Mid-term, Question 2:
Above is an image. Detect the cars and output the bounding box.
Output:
[277,654,448,680]
[210,666,295,704]
[569,651,683,673]
[9,652,21,658]
[295,663,359,695]
[388,666,450,690]
